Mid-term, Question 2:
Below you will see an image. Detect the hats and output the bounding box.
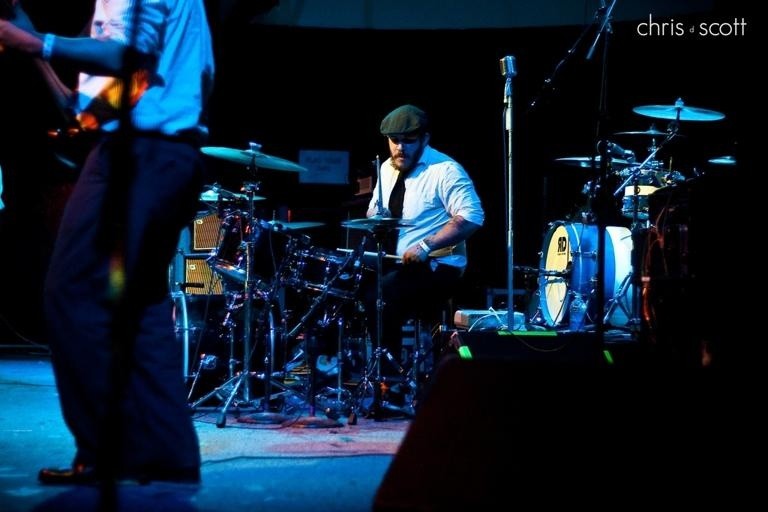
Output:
[380,105,425,134]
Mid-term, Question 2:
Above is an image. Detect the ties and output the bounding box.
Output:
[380,170,407,274]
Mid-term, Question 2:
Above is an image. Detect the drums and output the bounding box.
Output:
[620,185,660,220]
[538,219,635,330]
[174,295,274,407]
[205,210,289,294]
[298,250,365,300]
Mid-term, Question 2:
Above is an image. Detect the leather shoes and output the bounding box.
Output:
[39,459,94,486]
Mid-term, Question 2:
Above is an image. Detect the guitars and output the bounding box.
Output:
[1,1,93,170]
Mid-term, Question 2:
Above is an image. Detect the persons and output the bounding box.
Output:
[365,104,485,408]
[0,0,217,488]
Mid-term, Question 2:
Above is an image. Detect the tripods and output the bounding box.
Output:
[185,184,339,429]
[344,235,422,425]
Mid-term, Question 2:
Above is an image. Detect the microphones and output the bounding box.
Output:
[499,56,519,78]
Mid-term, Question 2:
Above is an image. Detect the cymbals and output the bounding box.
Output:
[633,98,725,121]
[708,156,735,164]
[199,140,310,172]
[555,153,643,168]
[267,219,325,229]
[341,212,418,229]
[198,189,267,201]
[615,127,682,136]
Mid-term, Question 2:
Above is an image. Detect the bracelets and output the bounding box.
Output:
[42,32,55,59]
[418,240,430,254]
